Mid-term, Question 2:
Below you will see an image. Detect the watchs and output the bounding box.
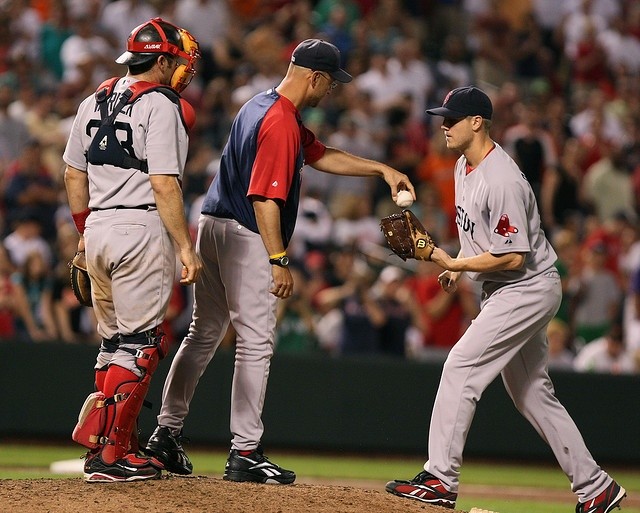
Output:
[269,257,290,266]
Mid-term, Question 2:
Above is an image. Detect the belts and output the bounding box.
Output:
[92,203,156,211]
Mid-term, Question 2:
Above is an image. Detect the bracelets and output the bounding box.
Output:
[71,209,92,235]
[269,251,287,259]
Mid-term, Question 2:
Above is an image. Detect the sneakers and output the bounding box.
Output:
[222,447,296,484]
[575,479,626,512]
[79,450,163,482]
[145,425,193,475]
[384,469,458,508]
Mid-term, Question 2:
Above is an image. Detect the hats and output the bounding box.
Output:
[424,84,492,119]
[291,39,353,83]
[115,17,201,93]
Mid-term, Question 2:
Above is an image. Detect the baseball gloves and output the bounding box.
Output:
[66,251,91,307]
[380,210,438,263]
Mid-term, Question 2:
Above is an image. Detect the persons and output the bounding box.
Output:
[82,18,204,485]
[143,36,417,486]
[384,84,629,509]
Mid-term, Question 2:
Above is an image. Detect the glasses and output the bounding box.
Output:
[316,72,340,90]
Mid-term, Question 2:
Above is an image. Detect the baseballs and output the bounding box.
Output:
[395,190,414,209]
